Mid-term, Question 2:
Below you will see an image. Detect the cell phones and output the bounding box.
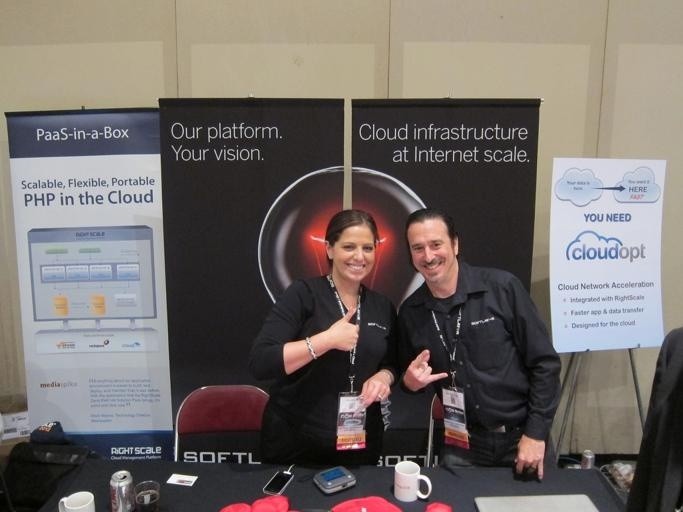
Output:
[263,470,294,495]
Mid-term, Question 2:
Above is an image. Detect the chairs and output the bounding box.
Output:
[173,386,268,463]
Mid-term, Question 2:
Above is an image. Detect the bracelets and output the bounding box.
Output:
[305,337,318,360]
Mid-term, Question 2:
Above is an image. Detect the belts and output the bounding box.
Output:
[469,423,526,432]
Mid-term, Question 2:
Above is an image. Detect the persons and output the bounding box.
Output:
[395,207,561,480]
[246,209,403,466]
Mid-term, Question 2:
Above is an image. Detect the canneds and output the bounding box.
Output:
[581,450,594,469]
[110,470,135,512]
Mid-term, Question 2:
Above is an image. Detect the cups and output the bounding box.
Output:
[392,459,434,505]
[57,490,96,512]
[133,480,163,512]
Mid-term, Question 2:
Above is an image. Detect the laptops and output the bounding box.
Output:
[474,494,599,512]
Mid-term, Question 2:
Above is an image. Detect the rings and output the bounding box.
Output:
[377,394,383,398]
[416,367,424,373]
